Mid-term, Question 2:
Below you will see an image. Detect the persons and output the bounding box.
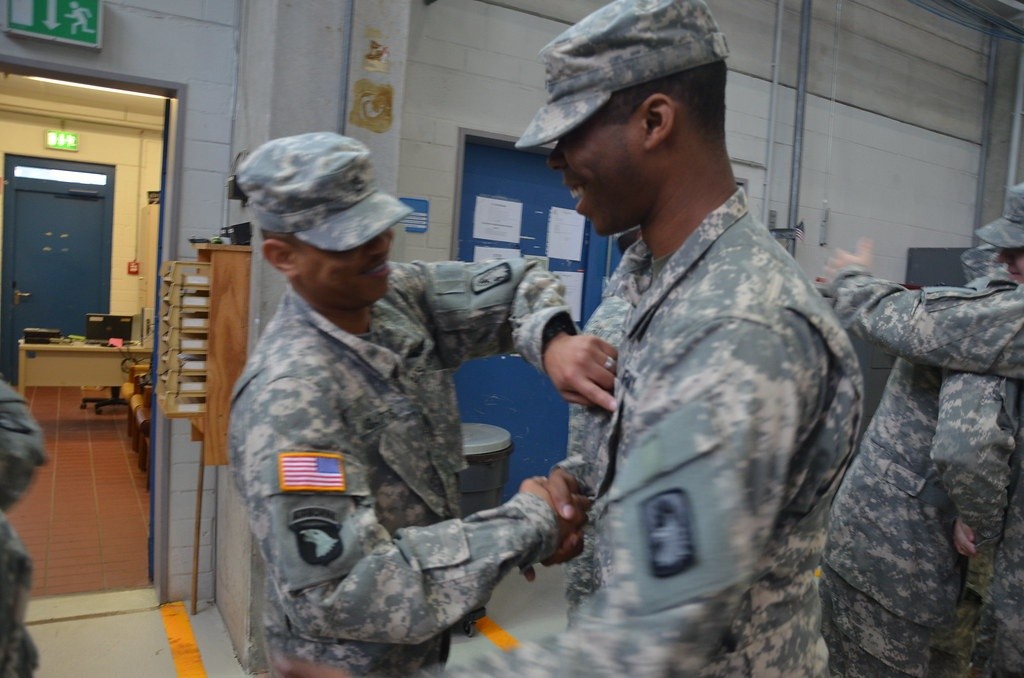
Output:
[225,130,621,678]
[271,0,864,678]
[0,377,51,678]
[815,181,1024,678]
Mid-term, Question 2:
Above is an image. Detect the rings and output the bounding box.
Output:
[604,355,613,370]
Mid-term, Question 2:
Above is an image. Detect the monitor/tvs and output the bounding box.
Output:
[84,313,134,346]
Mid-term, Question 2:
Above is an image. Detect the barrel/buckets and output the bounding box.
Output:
[460,422,515,519]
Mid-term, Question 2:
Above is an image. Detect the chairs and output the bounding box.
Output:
[118,364,153,491]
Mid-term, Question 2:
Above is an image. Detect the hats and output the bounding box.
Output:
[236,131,416,252]
[975,184,1024,251]
[516,0,731,151]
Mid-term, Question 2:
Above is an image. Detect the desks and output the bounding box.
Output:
[18,338,153,397]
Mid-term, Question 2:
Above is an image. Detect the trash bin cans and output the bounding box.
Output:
[453,420,516,638]
